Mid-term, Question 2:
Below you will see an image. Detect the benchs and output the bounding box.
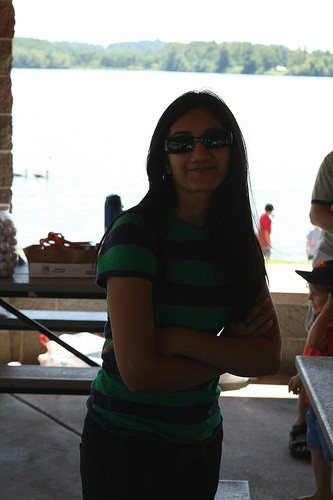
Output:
[0,308,107,396]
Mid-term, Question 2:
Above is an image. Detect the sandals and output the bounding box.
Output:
[288,422,310,459]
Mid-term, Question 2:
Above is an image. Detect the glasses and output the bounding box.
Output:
[163,131,233,154]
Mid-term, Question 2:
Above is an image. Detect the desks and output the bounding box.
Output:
[0,273,107,299]
[295,354,333,455]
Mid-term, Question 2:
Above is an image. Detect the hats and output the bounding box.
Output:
[295,259,332,284]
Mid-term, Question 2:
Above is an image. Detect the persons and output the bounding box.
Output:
[79,89,282,500]
[293,259,333,500]
[258,205,276,260]
[288,151,333,458]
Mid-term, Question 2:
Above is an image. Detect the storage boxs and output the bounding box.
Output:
[24,243,97,279]
